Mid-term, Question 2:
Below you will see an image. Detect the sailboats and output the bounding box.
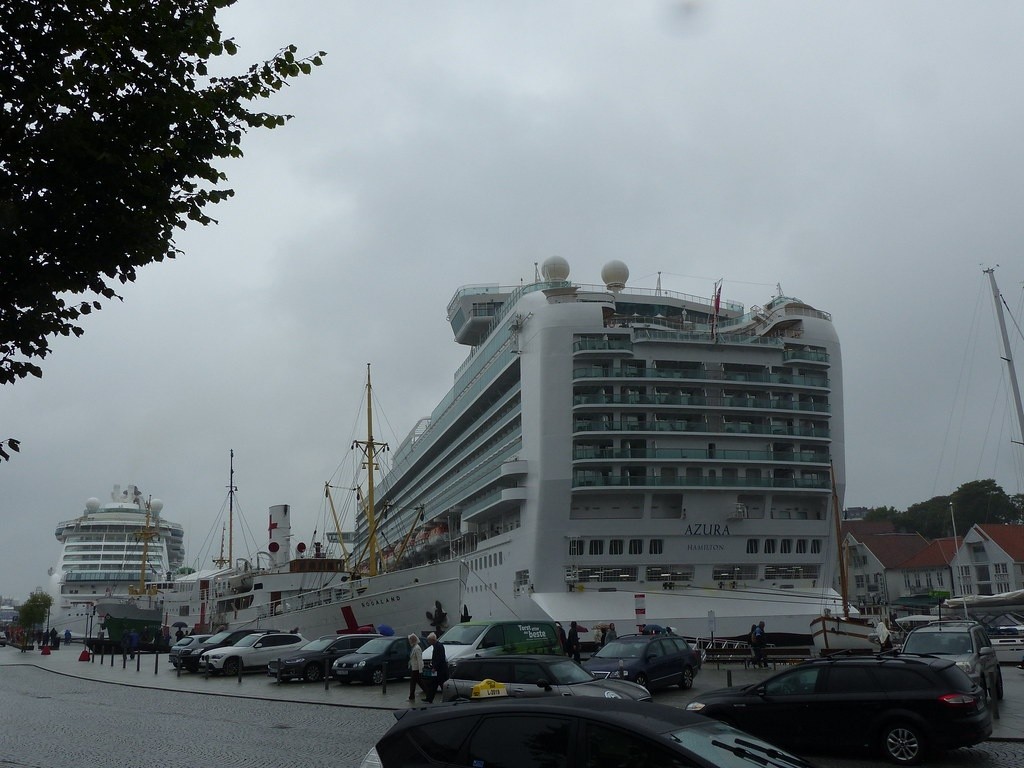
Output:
[83,362,471,655]
[811,459,905,658]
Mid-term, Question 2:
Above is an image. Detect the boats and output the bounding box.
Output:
[428,527,450,548]
[415,529,434,552]
[895,589,1024,666]
[380,552,398,569]
[393,538,415,559]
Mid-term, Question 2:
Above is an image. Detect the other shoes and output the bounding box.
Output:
[406,698,415,702]
[422,698,432,703]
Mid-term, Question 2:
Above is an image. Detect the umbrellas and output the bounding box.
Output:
[173,622,188,628]
[643,625,662,632]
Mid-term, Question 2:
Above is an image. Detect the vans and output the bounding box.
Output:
[407,620,564,692]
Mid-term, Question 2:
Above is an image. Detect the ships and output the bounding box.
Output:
[344,256,860,655]
[43,484,185,643]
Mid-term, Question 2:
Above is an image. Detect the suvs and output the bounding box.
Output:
[267,634,383,683]
[684,649,993,768]
[580,633,700,693]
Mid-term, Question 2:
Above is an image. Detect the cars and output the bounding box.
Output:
[169,635,214,669]
[359,678,811,768]
[178,629,279,673]
[198,632,311,677]
[331,636,431,686]
[898,620,1003,709]
[0,632,7,648]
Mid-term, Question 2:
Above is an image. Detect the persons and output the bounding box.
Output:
[37,628,57,645]
[568,622,580,664]
[601,623,617,645]
[555,622,566,641]
[748,621,771,670]
[435,626,444,639]
[642,624,673,635]
[176,627,196,642]
[420,632,449,704]
[121,629,139,659]
[65,630,70,641]
[405,633,427,702]
